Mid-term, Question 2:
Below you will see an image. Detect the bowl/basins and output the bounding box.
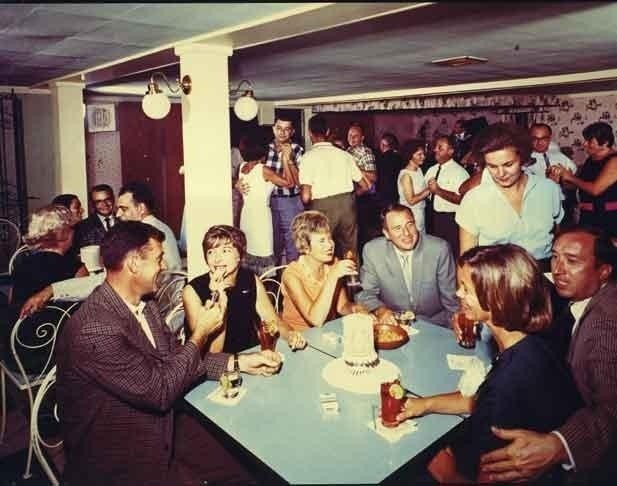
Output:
[374,324,408,350]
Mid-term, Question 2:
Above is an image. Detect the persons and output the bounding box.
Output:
[2,115,617,486]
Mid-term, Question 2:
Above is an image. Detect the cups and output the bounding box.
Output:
[221,368,242,399]
[259,321,275,351]
[459,309,475,348]
[393,311,411,332]
[344,253,361,285]
[381,384,402,427]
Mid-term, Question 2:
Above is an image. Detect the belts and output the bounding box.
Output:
[581,202,617,210]
[271,193,301,198]
[433,212,455,215]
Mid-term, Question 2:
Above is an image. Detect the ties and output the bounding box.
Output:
[402,255,411,298]
[430,165,441,211]
[564,303,575,353]
[104,217,110,233]
[543,153,550,169]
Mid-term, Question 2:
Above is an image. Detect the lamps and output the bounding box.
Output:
[142,72,191,119]
[230,79,258,122]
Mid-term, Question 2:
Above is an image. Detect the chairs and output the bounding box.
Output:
[259,264,288,312]
[29,362,60,486]
[155,270,188,314]
[164,302,185,346]
[0,297,78,479]
[1,218,21,302]
[7,244,32,306]
